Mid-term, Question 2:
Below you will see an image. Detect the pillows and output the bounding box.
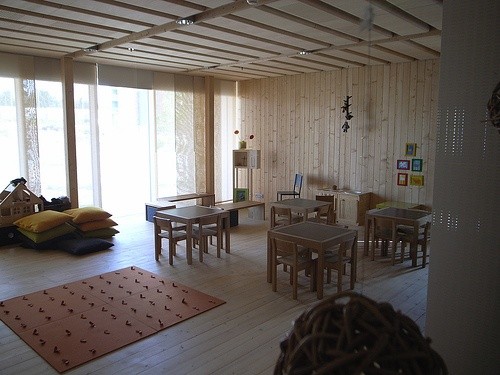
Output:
[13,207,120,255]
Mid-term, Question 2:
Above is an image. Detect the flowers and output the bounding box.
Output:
[234,130,254,141]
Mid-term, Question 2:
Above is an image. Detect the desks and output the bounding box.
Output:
[272,220,358,298]
[217,201,265,221]
[271,198,333,220]
[369,206,432,267]
[154,206,230,265]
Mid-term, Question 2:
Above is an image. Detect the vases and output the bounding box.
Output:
[239,141,246,149]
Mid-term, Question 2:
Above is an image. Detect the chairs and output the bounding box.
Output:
[151,173,433,299]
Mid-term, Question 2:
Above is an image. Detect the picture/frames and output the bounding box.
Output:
[409,175,424,186]
[397,160,410,170]
[234,188,249,203]
[406,142,416,157]
[412,159,422,172]
[397,173,408,186]
